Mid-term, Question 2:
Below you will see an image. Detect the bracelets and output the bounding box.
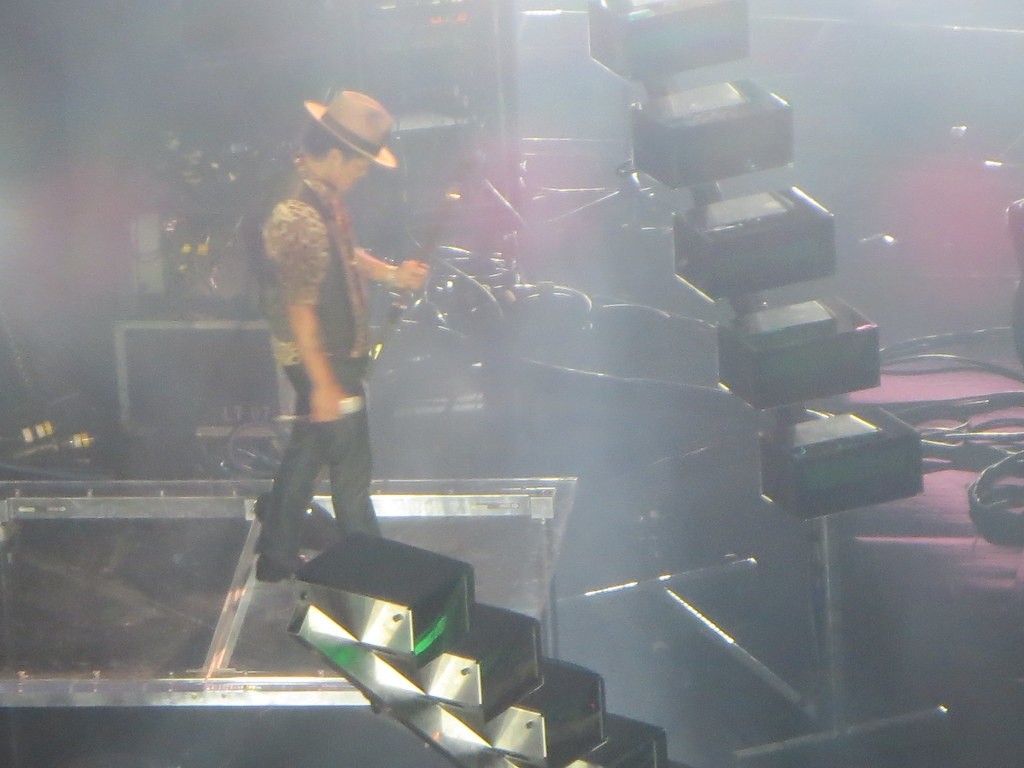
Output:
[385,265,398,288]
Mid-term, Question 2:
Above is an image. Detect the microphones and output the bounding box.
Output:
[272,397,362,424]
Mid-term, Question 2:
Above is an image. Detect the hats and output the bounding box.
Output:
[305,91,397,167]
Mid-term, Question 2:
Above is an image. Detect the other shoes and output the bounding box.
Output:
[257,555,308,582]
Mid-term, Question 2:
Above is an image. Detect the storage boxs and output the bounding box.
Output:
[110,285,297,442]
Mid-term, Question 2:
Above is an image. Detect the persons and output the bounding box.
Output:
[255,90,428,582]
